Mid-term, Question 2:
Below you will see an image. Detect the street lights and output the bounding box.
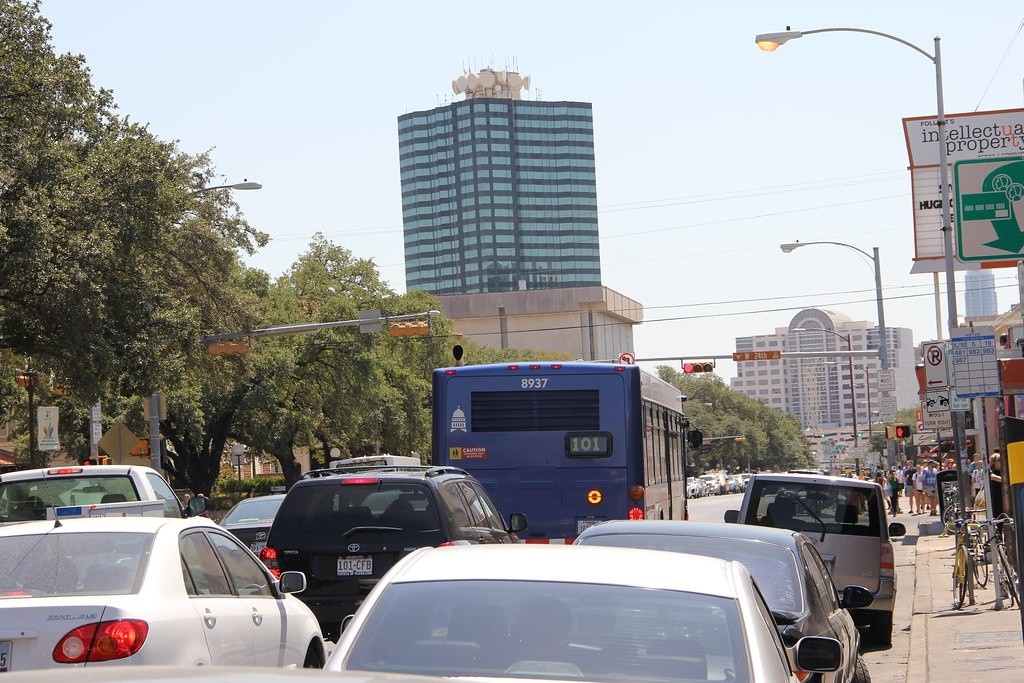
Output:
[789,326,862,478]
[22,363,38,469]
[232,445,246,499]
[823,361,872,444]
[754,26,962,532]
[779,239,896,482]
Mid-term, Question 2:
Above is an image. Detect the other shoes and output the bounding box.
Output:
[930,512,936,516]
[926,506,931,510]
[888,510,893,514]
[917,510,922,515]
[897,509,904,514]
[934,511,940,516]
[922,510,925,514]
[908,510,913,513]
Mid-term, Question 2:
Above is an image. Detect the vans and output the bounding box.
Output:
[329,455,423,509]
[1,464,209,526]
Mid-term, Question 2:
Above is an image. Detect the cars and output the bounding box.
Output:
[216,494,291,557]
[0,515,328,671]
[572,518,873,683]
[684,471,756,499]
[319,541,845,682]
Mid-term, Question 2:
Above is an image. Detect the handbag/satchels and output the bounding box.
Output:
[884,483,893,496]
[974,484,986,509]
[899,483,904,490]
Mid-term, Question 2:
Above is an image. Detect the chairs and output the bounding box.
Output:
[760,496,805,523]
[826,503,868,529]
[0,494,373,593]
[410,635,706,681]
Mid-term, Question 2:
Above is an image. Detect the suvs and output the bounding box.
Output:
[722,472,907,647]
[256,468,530,658]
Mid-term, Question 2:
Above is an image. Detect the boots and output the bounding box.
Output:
[886,501,891,510]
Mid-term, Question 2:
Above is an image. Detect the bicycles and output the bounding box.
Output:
[933,489,1018,610]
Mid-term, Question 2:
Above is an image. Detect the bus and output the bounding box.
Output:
[431,361,702,546]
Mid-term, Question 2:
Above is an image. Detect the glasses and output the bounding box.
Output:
[951,462,954,463]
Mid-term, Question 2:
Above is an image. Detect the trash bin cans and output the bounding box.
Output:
[936,470,957,523]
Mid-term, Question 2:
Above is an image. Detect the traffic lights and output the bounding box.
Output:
[83,460,96,466]
[896,426,910,438]
[851,434,862,438]
[681,362,715,374]
[813,434,825,438]
[885,426,895,441]
[105,458,113,465]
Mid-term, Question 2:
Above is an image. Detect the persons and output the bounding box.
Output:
[181,492,210,518]
[872,447,1005,542]
